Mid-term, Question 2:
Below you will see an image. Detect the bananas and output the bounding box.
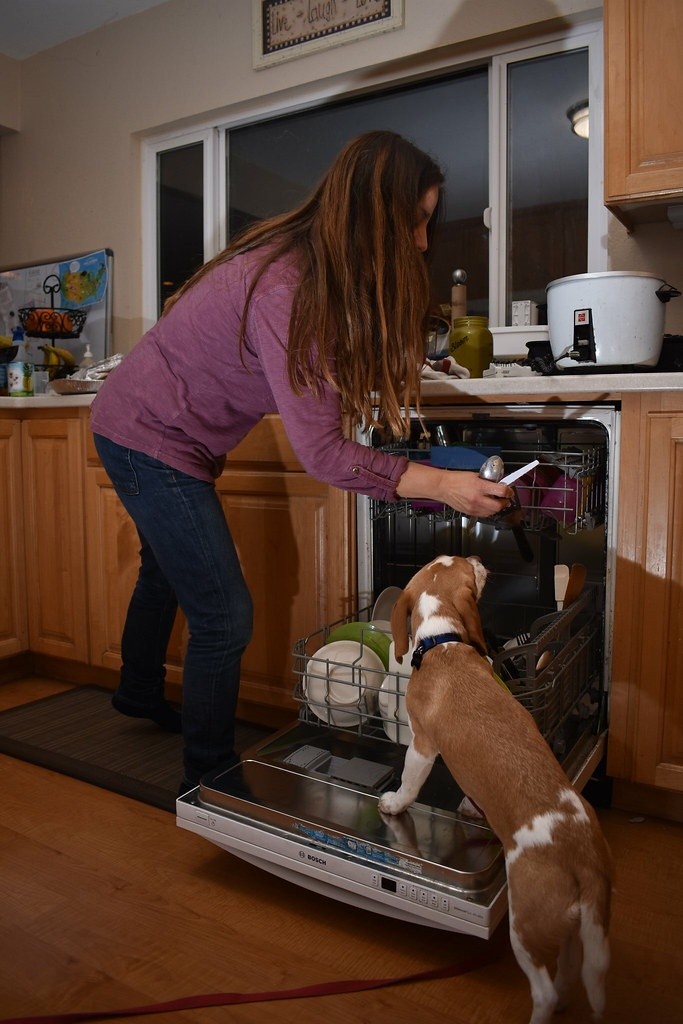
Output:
[37,344,76,382]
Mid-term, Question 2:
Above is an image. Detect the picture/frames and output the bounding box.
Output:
[250,0,404,72]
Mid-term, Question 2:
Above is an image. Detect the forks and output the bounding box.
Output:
[515,632,533,650]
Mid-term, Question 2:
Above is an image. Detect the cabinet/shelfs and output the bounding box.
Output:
[0,389,683,827]
[604,0,683,236]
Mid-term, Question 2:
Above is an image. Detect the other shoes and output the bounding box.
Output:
[113,683,184,732]
[182,742,239,783]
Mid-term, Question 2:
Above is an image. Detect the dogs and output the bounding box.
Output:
[378,554,615,1023]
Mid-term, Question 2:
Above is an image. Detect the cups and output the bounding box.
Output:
[540,475,581,525]
[32,371,49,396]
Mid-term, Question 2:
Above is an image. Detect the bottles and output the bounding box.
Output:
[7,328,35,396]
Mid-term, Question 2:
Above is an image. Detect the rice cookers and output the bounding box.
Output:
[535,271,683,375]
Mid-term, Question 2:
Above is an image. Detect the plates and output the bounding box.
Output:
[302,585,416,747]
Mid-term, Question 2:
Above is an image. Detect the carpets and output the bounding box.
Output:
[0,685,275,815]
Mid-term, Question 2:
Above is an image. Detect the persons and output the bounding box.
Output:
[88,131,515,785]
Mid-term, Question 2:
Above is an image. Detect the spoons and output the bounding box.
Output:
[534,650,559,672]
[465,455,504,538]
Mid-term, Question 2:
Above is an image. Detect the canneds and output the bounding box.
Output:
[6,361,35,397]
[447,314,493,378]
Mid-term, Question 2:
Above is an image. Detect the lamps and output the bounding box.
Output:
[567,98,589,138]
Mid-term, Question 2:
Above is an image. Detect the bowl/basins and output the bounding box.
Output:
[511,467,547,519]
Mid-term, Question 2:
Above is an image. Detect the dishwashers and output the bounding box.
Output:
[170,402,620,941]
